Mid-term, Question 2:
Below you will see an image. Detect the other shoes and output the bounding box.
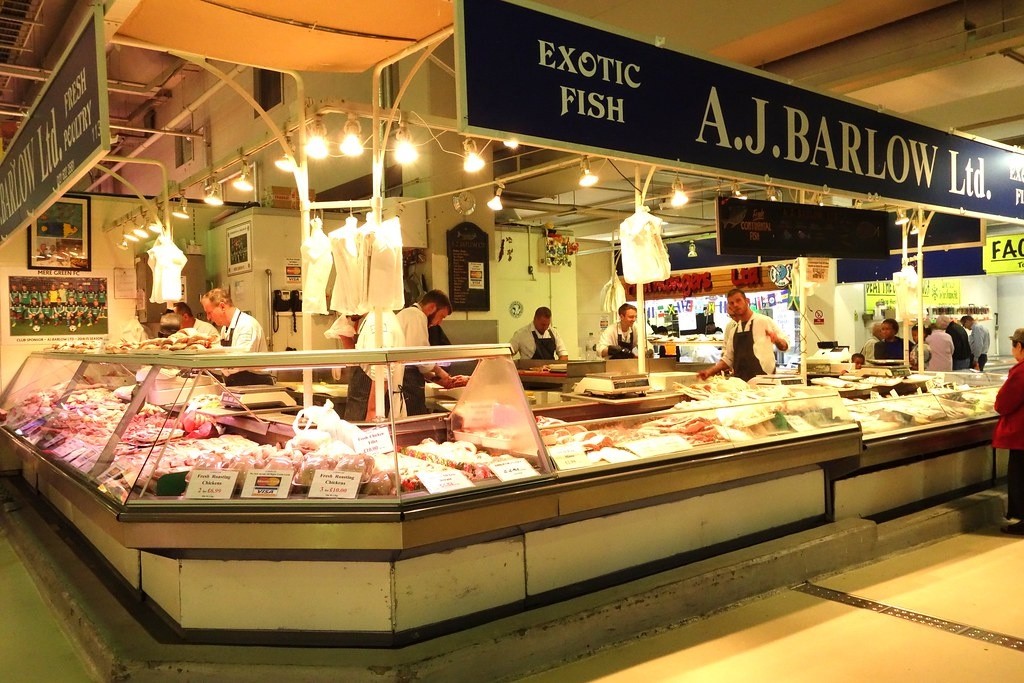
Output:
[11,320,98,327]
[1000,520,1024,535]
[98,312,107,318]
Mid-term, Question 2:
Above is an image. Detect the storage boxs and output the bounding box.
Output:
[264,185,315,209]
[649,372,699,391]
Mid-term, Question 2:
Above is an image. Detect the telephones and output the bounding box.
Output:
[291,290,302,312]
[273,289,290,312]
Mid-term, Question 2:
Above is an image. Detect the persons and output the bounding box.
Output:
[596,303,668,359]
[336,289,465,422]
[697,289,791,381]
[203,291,269,353]
[10,283,107,327]
[509,307,568,363]
[171,302,223,347]
[993,329,1024,537]
[841,316,991,371]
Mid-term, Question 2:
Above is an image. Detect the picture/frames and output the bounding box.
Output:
[28,194,92,271]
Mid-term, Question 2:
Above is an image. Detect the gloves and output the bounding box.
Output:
[608,345,635,358]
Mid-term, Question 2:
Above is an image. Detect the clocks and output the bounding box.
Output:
[452,188,476,215]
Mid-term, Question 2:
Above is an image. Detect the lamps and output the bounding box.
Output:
[688,243,697,257]
[233,156,253,191]
[766,186,779,201]
[578,160,599,187]
[463,138,484,173]
[895,209,909,225]
[487,188,504,210]
[729,181,747,200]
[172,198,189,218]
[205,174,224,205]
[273,113,418,173]
[671,178,688,205]
[116,207,163,250]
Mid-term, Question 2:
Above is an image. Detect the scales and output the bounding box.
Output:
[747,374,804,387]
[221,384,296,410]
[859,359,911,379]
[573,372,650,398]
